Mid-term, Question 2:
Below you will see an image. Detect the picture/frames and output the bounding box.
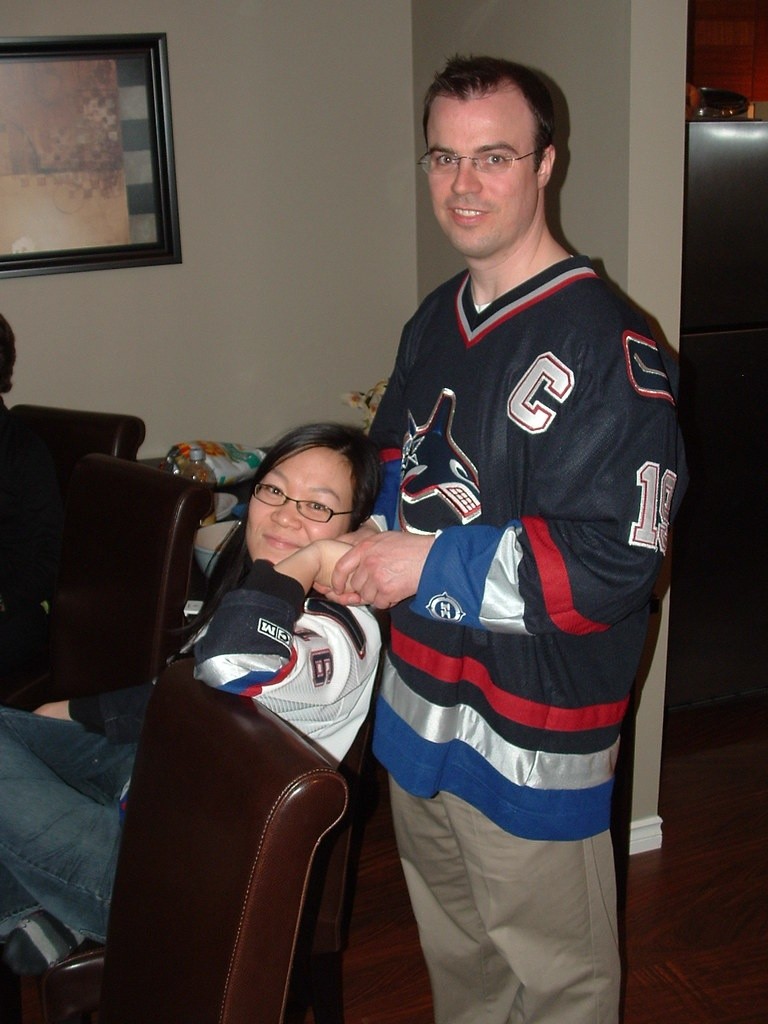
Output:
[0,31,182,279]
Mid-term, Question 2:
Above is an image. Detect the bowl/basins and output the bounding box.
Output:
[193,520,246,577]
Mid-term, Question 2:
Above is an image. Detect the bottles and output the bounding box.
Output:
[183,446,217,533]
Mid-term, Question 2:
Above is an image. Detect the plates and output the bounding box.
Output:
[216,493,239,520]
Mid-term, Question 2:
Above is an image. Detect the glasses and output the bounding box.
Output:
[417,147,538,176]
[252,482,353,523]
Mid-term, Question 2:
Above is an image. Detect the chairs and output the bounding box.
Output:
[0,454,215,710]
[102,677,350,1024]
[0,405,145,681]
[15,606,391,1024]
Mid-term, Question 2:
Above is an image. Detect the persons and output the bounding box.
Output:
[313,53,692,1024]
[0,423,384,1024]
[0,316,54,692]
